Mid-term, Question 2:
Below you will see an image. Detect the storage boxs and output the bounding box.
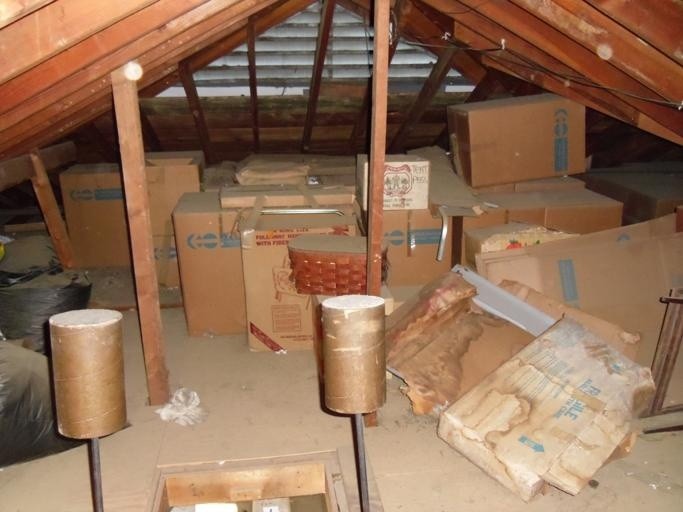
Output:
[451,178,592,192]
[360,205,453,304]
[174,189,356,337]
[146,449,350,510]
[237,207,358,353]
[446,94,589,189]
[58,149,205,268]
[597,172,681,227]
[462,218,581,267]
[356,153,431,212]
[455,189,546,268]
[516,190,625,237]
